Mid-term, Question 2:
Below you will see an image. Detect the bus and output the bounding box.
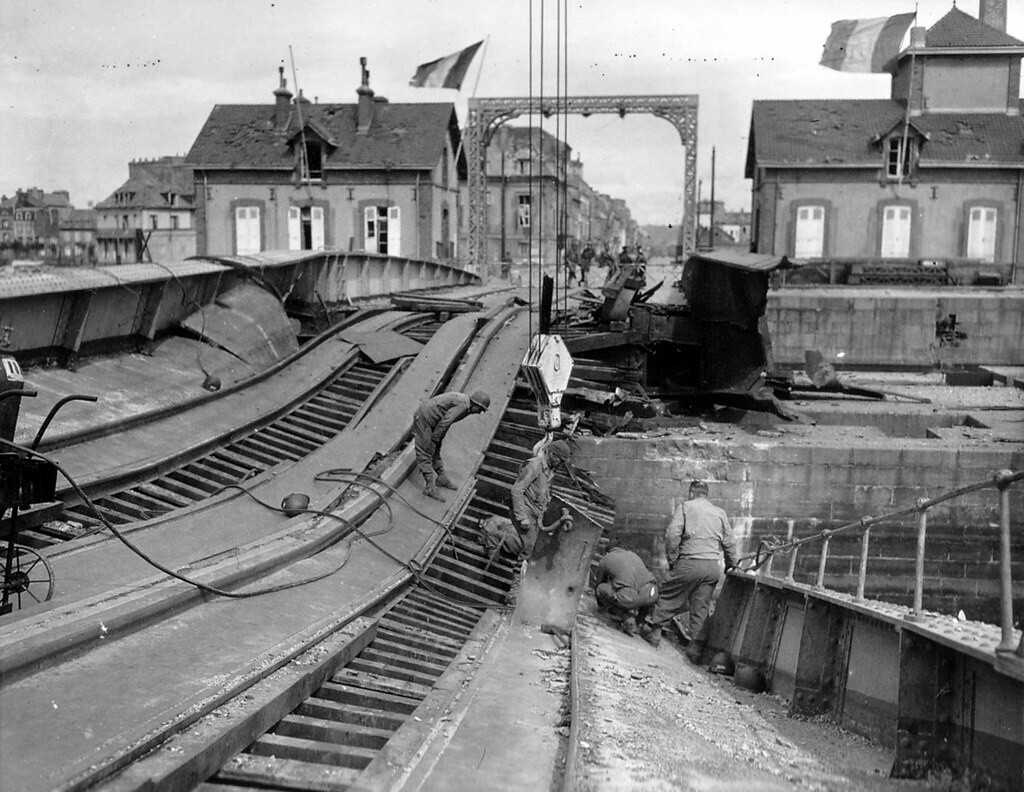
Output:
[11,260,45,274]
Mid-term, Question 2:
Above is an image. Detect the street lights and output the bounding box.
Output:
[709,146,716,247]
[696,179,703,247]
[499,126,514,278]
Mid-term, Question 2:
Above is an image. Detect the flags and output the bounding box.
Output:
[408,39,486,92]
[818,11,917,75]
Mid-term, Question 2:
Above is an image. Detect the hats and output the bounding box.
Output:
[550,440,571,461]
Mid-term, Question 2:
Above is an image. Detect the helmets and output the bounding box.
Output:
[470,391,491,412]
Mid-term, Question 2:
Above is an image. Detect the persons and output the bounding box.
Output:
[639,480,741,663]
[594,537,659,638]
[412,390,491,503]
[564,242,646,289]
[472,439,571,563]
[499,251,513,282]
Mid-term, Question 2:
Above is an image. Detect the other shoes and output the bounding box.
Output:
[640,628,660,647]
[624,623,636,633]
[436,478,458,490]
[687,644,702,663]
[423,488,446,502]
